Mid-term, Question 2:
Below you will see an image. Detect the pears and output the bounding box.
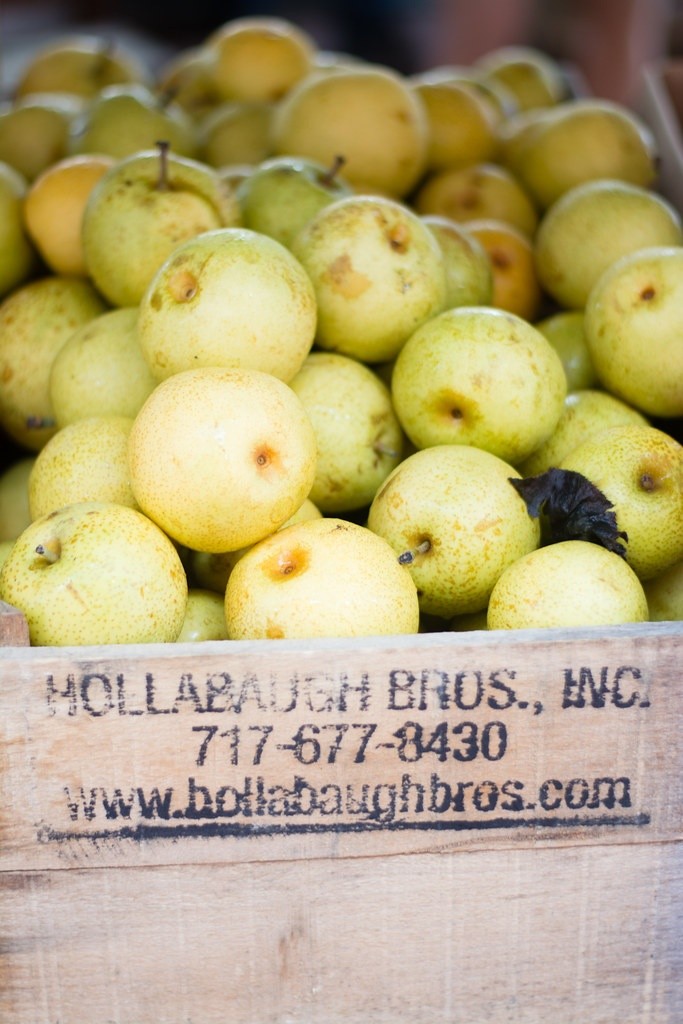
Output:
[0,18,683,648]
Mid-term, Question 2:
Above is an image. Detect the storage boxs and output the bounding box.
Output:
[0,66,683,1023]
